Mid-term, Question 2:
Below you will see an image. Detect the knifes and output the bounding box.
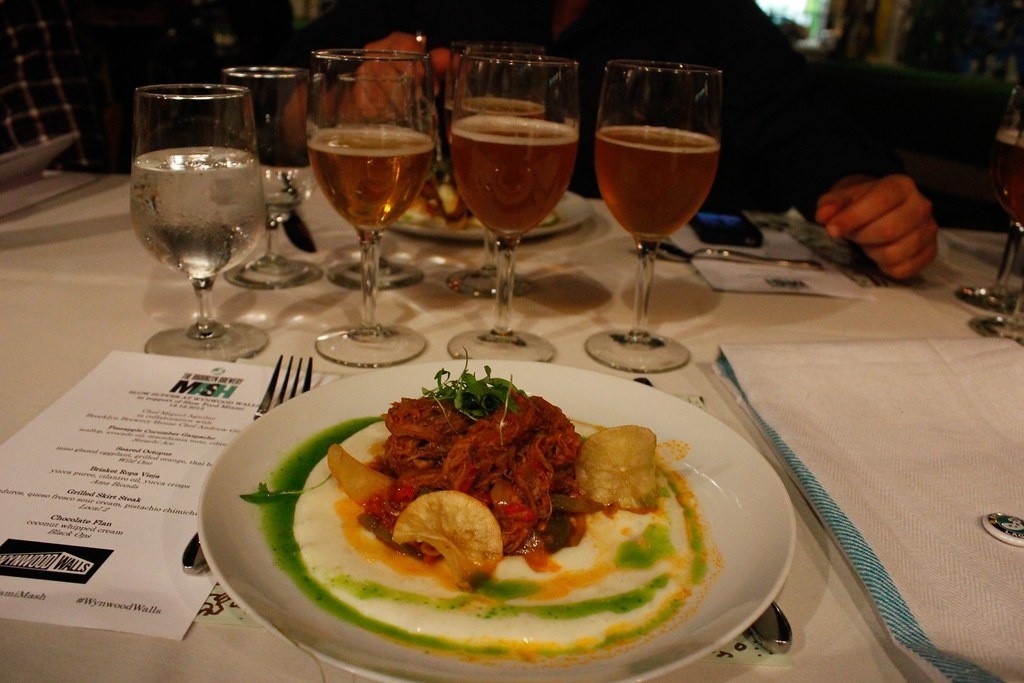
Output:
[632,376,793,654]
[281,210,317,254]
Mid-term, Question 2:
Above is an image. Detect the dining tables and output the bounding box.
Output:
[0,169,1024,683]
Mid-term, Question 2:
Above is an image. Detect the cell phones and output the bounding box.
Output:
[690,203,764,248]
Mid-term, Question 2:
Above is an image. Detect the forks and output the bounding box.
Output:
[181,354,312,576]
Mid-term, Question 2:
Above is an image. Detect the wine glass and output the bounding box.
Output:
[446,41,546,296]
[446,54,580,362]
[585,59,722,371]
[131,83,270,360]
[221,66,323,288]
[312,73,424,290]
[968,290,1024,346]
[953,89,1024,315]
[305,49,434,365]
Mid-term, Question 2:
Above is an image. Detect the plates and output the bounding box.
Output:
[197,360,796,683]
[391,192,594,245]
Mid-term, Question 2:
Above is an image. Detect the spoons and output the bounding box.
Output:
[642,240,824,272]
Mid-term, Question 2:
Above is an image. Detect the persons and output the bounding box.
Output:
[898,0,982,72]
[819,9,883,65]
[0,0,294,175]
[269,0,939,280]
